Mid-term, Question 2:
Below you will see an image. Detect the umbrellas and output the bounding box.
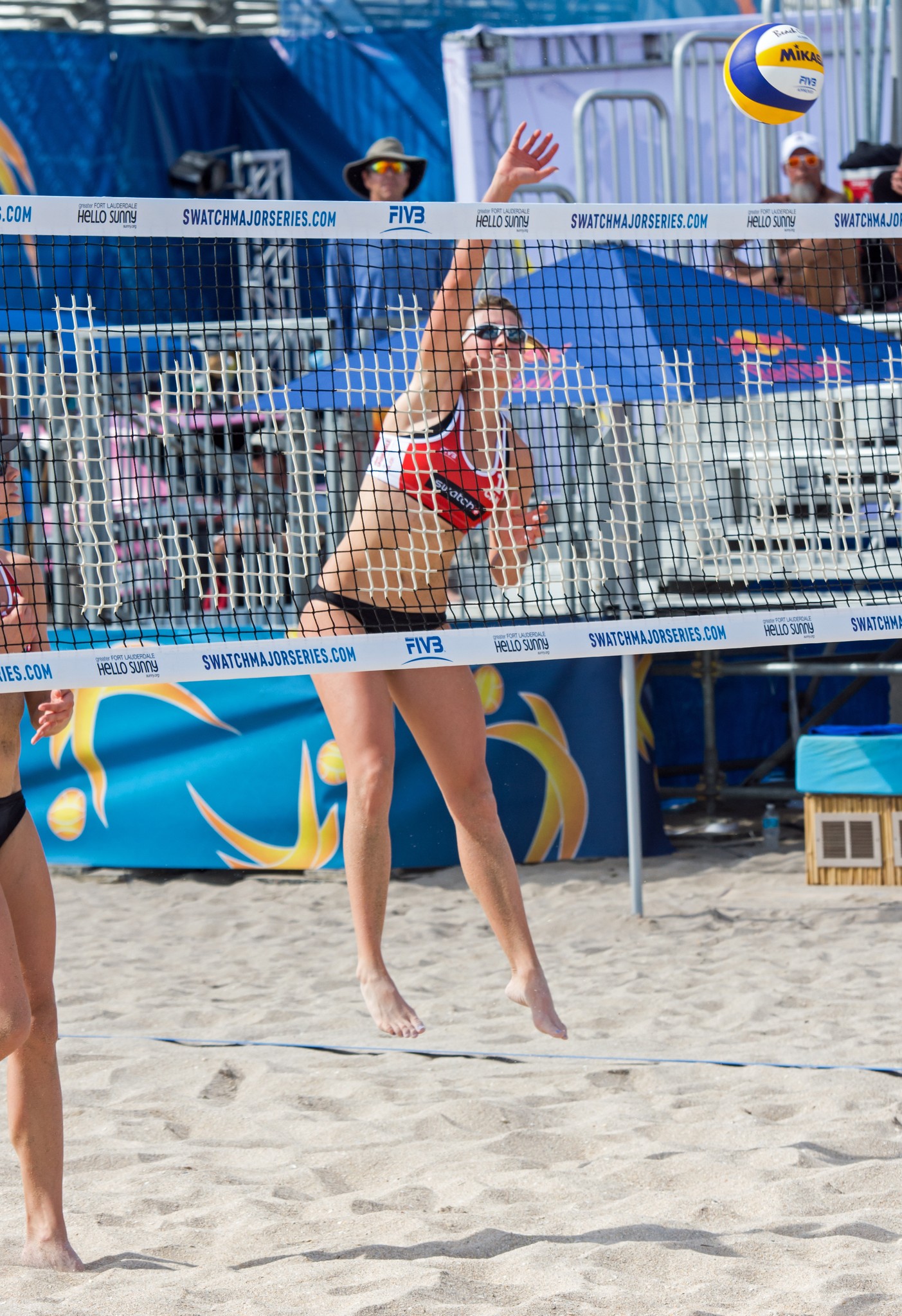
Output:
[229,237,902,918]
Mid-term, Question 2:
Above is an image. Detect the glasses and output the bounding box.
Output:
[370,160,407,174]
[462,323,528,348]
[250,449,259,459]
[0,455,8,475]
[789,154,817,165]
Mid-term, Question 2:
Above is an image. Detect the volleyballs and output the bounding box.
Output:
[722,23,825,125]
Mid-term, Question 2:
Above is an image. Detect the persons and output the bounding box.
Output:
[0,433,88,1274]
[297,120,569,1041]
[327,137,454,361]
[714,130,902,316]
[213,429,343,564]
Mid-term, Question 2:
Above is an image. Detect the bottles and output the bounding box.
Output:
[762,804,780,852]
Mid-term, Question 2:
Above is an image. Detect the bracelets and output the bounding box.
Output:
[771,259,784,277]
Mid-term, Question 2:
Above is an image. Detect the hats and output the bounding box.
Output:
[0,429,23,454]
[253,428,286,451]
[343,137,426,198]
[781,131,823,163]
